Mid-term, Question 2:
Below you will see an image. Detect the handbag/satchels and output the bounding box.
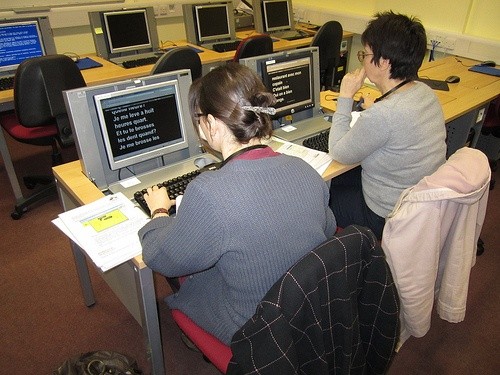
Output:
[55,350,142,375]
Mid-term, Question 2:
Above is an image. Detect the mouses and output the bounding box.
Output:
[446,76,460,83]
[194,157,216,168]
[324,115,332,122]
[71,57,78,62]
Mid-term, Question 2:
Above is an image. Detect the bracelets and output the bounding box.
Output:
[152,208,169,218]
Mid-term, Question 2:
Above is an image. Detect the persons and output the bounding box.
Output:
[139,62,335,363]
[330,10,450,243]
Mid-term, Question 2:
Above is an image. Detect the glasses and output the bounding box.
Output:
[357,50,381,62]
[193,112,216,124]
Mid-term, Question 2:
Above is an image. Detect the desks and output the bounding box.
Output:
[50,57,500,375]
[0,22,356,202]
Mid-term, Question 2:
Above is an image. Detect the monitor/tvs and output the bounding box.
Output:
[89,6,159,61]
[180,0,237,46]
[0,18,58,72]
[251,0,295,34]
[63,68,203,192]
[238,47,325,131]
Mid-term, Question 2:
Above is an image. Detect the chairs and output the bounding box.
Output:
[0,55,87,220]
[232,35,275,62]
[172,148,493,375]
[310,20,344,90]
[150,47,204,83]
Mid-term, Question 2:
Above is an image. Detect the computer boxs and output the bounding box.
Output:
[327,36,352,87]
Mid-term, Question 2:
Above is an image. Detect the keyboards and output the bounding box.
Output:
[283,31,315,40]
[213,40,243,52]
[134,161,223,217]
[0,76,15,91]
[123,57,161,68]
[303,129,329,153]
